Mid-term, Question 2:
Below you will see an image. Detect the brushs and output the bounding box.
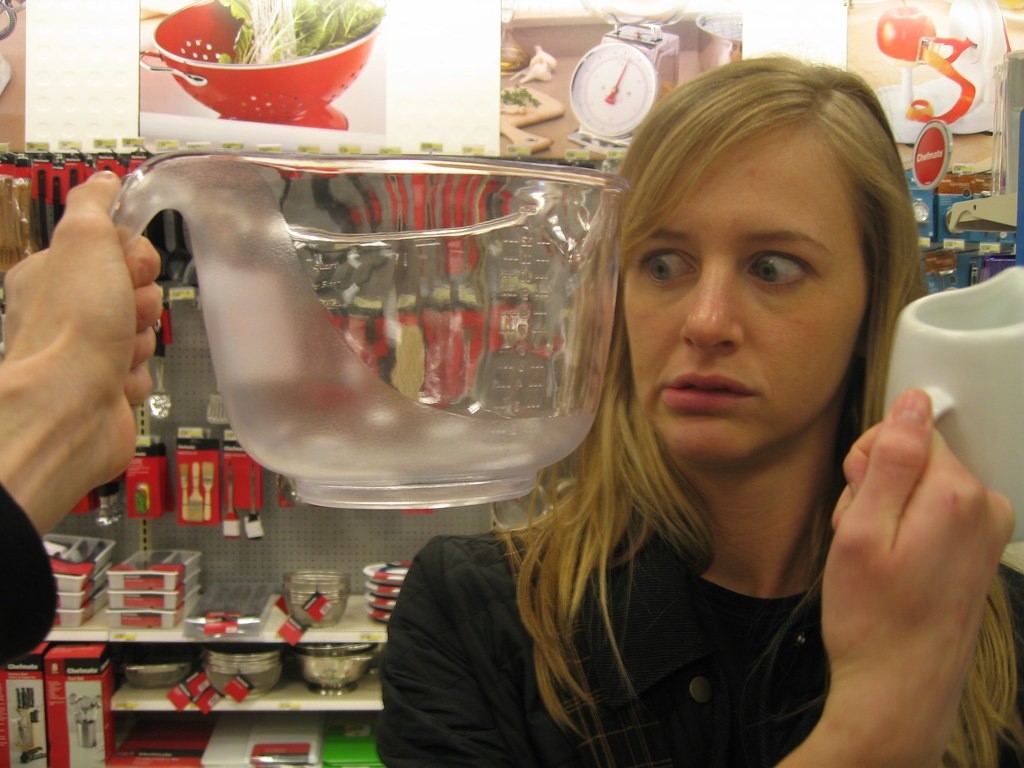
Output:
[177,462,215,523]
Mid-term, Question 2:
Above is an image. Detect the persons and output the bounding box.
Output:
[373,55,1024,768]
[1,171,164,664]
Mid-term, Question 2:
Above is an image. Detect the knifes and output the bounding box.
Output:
[14,686,39,722]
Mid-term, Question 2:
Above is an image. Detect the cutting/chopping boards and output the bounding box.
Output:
[500,85,564,154]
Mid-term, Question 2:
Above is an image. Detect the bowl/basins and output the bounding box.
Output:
[282,570,351,626]
[121,658,194,689]
[199,646,282,701]
[140,0,386,132]
[296,642,377,696]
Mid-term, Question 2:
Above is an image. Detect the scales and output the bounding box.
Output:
[566,0,684,160]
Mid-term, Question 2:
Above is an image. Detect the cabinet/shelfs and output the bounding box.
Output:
[110,595,393,768]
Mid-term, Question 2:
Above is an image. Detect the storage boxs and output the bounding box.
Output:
[41,534,203,627]
[0,643,115,768]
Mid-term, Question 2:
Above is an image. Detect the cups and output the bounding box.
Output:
[76,719,97,747]
[883,265,1024,542]
[102,150,633,510]
